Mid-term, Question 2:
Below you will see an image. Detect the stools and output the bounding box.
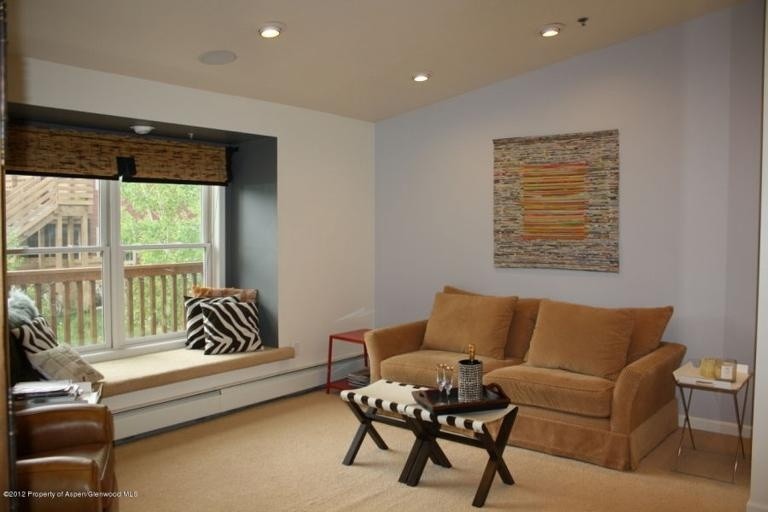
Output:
[340,379,518,507]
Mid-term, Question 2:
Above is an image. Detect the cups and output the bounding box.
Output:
[458,359,483,403]
[700,357,737,382]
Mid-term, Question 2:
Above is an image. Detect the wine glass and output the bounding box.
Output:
[437,363,454,404]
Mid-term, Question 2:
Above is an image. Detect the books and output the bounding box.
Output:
[11,378,83,407]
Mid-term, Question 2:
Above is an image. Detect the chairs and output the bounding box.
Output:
[14,403,114,512]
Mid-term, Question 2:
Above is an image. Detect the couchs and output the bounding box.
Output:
[363,286,687,471]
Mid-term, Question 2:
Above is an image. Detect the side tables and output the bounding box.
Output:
[672,361,752,484]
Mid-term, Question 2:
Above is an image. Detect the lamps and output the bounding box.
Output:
[256,21,287,40]
[130,125,154,135]
[536,23,565,40]
[409,70,432,84]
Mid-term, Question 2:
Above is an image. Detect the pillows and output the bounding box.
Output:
[8,285,104,385]
[418,286,673,382]
[182,285,264,355]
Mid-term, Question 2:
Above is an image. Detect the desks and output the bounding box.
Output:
[326,329,373,394]
